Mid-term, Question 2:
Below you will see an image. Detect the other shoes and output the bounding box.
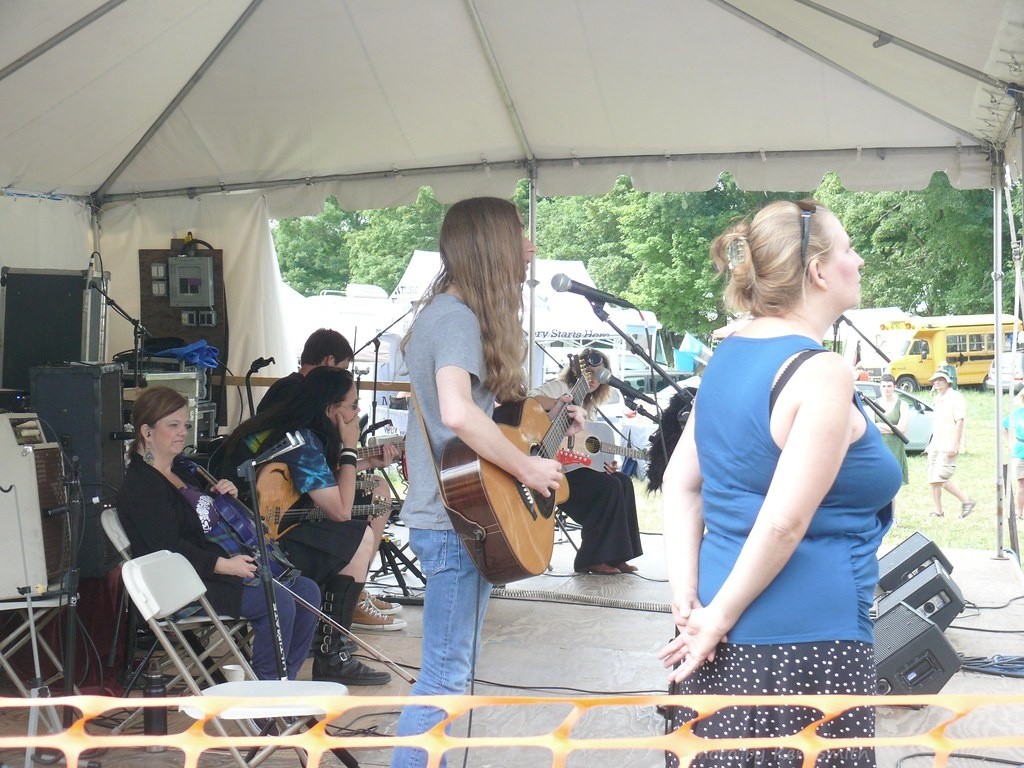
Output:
[929,512,943,519]
[250,717,292,736]
[960,495,976,518]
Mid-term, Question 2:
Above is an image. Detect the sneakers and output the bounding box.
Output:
[360,588,403,615]
[306,631,358,659]
[350,596,407,631]
[311,641,391,685]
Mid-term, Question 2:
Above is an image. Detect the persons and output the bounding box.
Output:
[925,366,978,522]
[529,349,642,576]
[116,385,321,736]
[207,364,390,686]
[655,197,902,767]
[1001,404,1024,522]
[389,197,562,768]
[258,329,406,633]
[866,375,909,529]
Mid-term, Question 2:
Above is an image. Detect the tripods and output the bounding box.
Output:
[235,429,361,768]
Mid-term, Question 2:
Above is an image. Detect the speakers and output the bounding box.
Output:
[868,529,966,711]
[0,412,77,600]
[29,361,128,579]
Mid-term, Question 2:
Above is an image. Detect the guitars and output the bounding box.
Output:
[356,439,405,458]
[557,420,648,476]
[399,433,591,485]
[439,351,595,586]
[251,460,392,542]
[355,474,382,498]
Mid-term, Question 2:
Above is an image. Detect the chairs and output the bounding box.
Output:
[121,551,361,768]
[99,506,299,736]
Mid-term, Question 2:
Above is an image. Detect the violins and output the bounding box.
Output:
[173,452,291,580]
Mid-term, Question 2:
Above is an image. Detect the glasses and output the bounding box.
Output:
[334,400,358,410]
[794,200,817,282]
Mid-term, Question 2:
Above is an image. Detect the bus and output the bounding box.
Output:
[890,322,1022,392]
[306,284,713,435]
[842,307,922,382]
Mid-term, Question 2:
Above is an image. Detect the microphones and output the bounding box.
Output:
[46,496,107,515]
[595,367,656,406]
[82,257,95,312]
[551,272,635,310]
[110,431,135,440]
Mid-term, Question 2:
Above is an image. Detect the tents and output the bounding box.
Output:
[2,3,1023,572]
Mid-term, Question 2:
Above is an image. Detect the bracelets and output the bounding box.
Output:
[339,448,356,467]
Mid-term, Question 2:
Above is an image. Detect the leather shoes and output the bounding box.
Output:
[575,562,620,574]
[612,561,638,573]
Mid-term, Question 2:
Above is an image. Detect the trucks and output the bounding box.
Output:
[986,329,1024,390]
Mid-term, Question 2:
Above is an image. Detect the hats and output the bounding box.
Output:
[927,369,953,387]
